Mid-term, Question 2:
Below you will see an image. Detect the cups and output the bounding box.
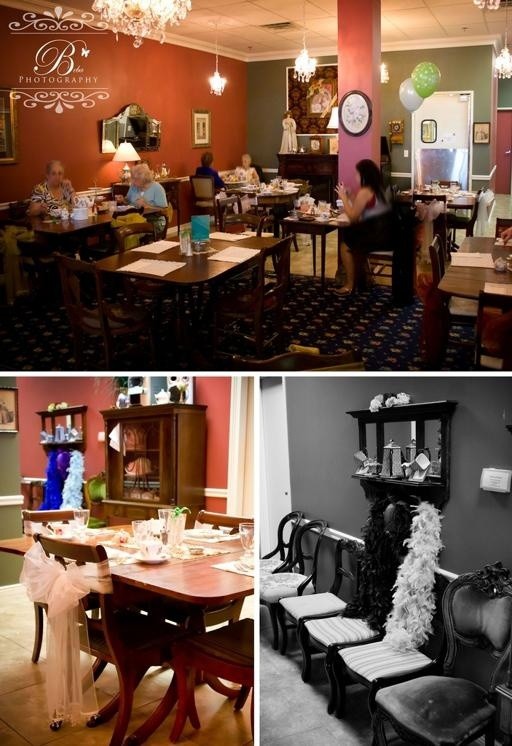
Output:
[158,508,174,545]
[107,200,117,217]
[168,512,187,546]
[74,509,90,544]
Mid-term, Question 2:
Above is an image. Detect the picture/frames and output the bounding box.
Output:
[338,91,372,137]
[0,88,17,166]
[0,387,20,433]
[167,376,195,404]
[310,136,323,153]
[192,109,212,149]
[473,122,490,144]
[285,64,339,137]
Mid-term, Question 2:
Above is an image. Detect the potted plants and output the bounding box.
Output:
[114,377,130,408]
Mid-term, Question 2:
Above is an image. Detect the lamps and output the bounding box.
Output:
[92,0,191,48]
[293,0,317,83]
[327,106,339,129]
[495,1,511,80]
[208,18,227,96]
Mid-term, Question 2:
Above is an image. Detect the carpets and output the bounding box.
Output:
[0,247,476,372]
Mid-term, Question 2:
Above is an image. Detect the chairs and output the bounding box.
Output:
[189,176,220,229]
[259,518,328,650]
[22,508,84,663]
[84,473,105,529]
[278,537,365,679]
[52,252,154,372]
[372,564,512,746]
[333,566,448,745]
[217,196,242,234]
[412,193,452,262]
[232,348,365,371]
[169,619,255,746]
[429,235,480,364]
[209,233,295,363]
[298,550,400,714]
[8,201,35,220]
[449,183,489,238]
[115,223,196,343]
[475,290,512,371]
[221,215,270,238]
[431,180,461,242]
[259,510,303,575]
[16,240,78,321]
[286,179,309,196]
[196,510,255,627]
[250,201,299,284]
[346,202,417,302]
[32,532,201,746]
[494,217,511,239]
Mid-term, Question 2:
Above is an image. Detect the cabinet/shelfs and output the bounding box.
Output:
[100,402,208,530]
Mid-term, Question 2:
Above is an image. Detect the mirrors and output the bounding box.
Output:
[344,399,457,513]
[36,404,87,456]
[101,104,161,154]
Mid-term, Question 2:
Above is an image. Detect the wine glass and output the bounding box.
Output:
[239,523,254,573]
[131,518,147,547]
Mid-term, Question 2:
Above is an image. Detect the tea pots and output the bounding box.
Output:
[378,439,405,479]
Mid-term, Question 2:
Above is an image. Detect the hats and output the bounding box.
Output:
[124,457,155,476]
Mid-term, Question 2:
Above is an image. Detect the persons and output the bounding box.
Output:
[194,151,226,224]
[29,160,78,219]
[338,159,392,298]
[117,165,173,250]
[232,156,260,191]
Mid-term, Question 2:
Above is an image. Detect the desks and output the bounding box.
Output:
[111,177,182,237]
[277,153,338,208]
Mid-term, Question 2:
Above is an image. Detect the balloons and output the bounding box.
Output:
[412,61,442,102]
[400,78,426,112]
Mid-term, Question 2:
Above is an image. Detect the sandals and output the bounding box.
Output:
[330,285,354,298]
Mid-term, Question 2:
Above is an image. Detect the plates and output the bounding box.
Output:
[134,551,170,564]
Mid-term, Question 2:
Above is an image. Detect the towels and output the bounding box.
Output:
[179,545,231,560]
[114,258,187,276]
[183,533,243,543]
[130,238,181,255]
[103,544,144,569]
[450,251,495,269]
[86,527,116,537]
[241,229,275,238]
[108,422,127,457]
[495,236,511,247]
[483,281,512,296]
[211,556,255,579]
[207,246,261,265]
[209,230,251,242]
[335,212,349,222]
